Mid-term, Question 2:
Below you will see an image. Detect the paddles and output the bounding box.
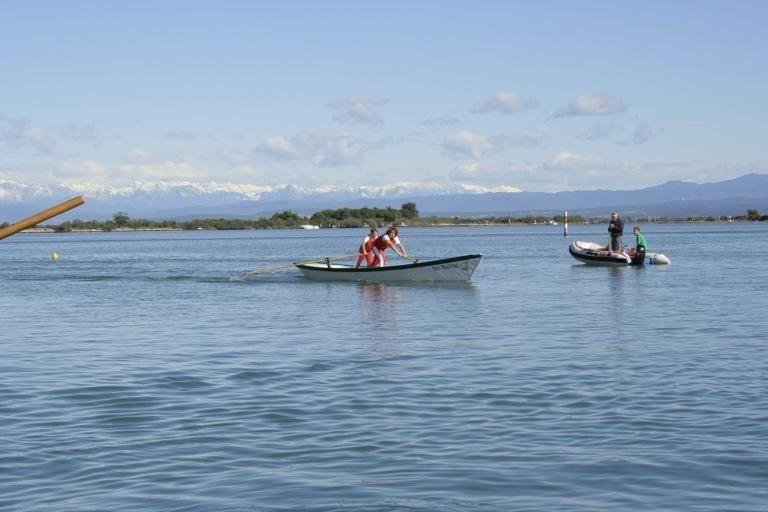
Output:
[241,252,373,274]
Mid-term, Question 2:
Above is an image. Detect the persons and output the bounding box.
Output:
[608,213,624,251]
[355,228,378,267]
[370,226,407,267]
[633,227,646,257]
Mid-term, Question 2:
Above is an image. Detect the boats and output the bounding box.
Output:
[292,254,482,283]
[569,240,670,266]
